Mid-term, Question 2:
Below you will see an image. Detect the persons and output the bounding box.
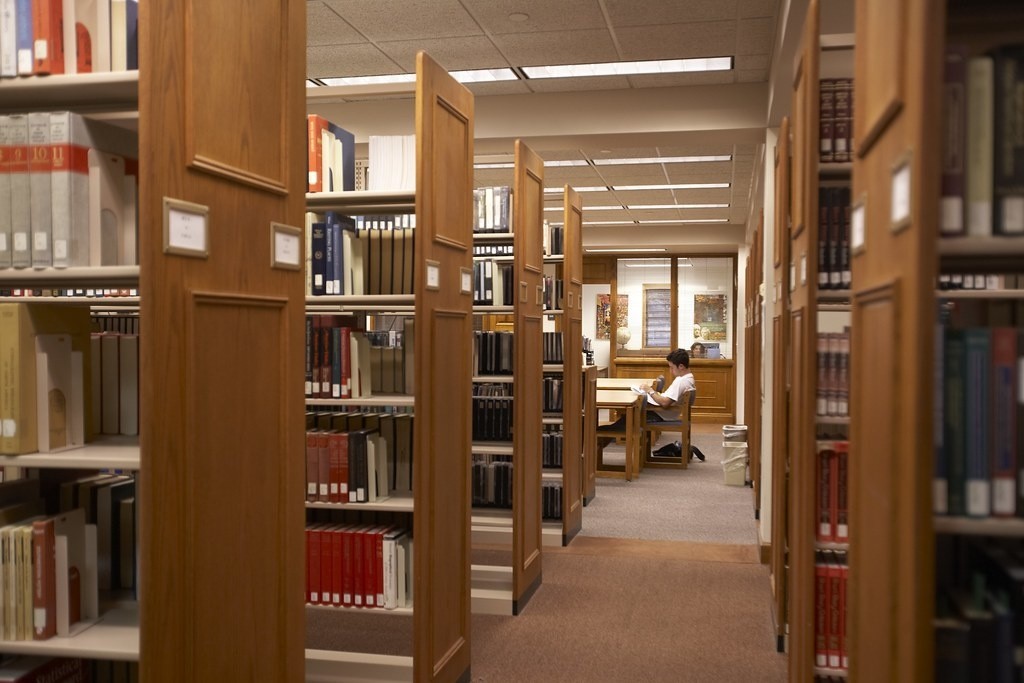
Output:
[599,349,696,447]
[691,343,704,358]
[694,323,710,341]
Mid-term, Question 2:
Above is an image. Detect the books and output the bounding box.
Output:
[0,0,563,683]
[816,30,1024,675]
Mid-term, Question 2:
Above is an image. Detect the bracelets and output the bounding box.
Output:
[649,390,656,396]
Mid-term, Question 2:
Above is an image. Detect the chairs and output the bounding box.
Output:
[613,378,662,447]
[642,389,696,471]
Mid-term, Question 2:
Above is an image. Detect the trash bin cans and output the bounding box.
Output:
[722,424,748,442]
[721,441,749,487]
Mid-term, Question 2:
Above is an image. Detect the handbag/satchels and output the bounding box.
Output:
[652,441,706,461]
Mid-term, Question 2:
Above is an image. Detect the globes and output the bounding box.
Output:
[616,327,632,351]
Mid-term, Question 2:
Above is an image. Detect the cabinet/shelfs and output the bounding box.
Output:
[770,0,1024,683]
[543,183,583,549]
[0,0,311,683]
[582,365,598,507]
[307,51,477,683]
[471,138,548,615]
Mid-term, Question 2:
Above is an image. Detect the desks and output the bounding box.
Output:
[596,378,657,447]
[594,390,642,482]
